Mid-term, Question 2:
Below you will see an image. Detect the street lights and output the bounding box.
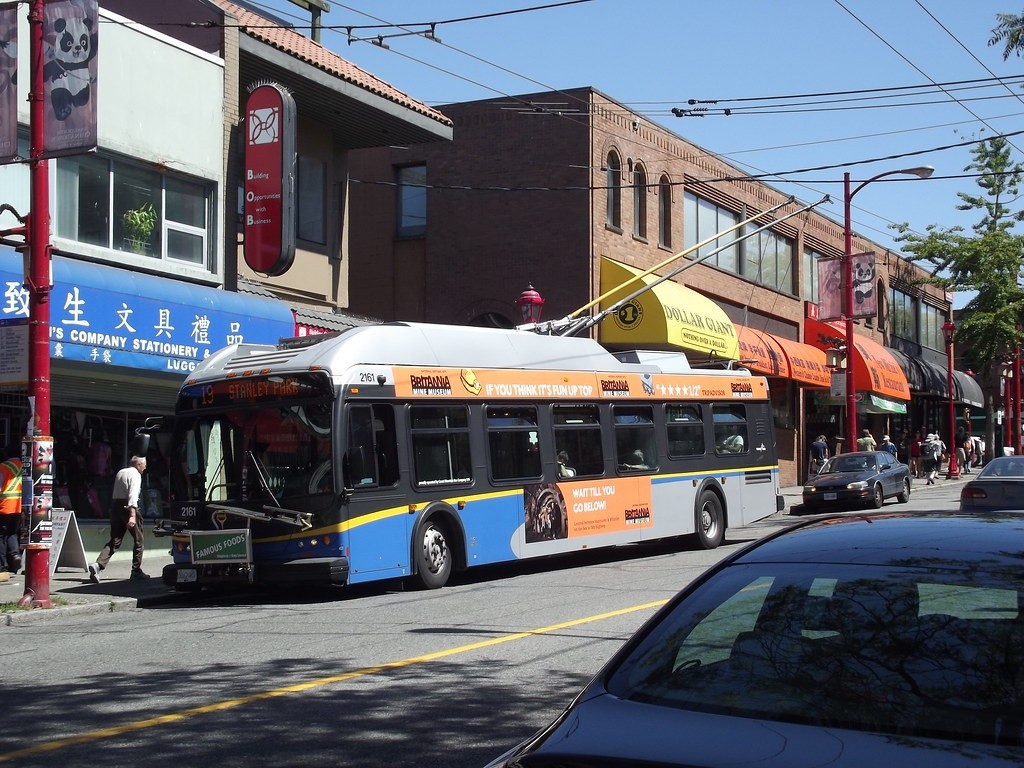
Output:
[843,165,936,451]
[940,318,960,477]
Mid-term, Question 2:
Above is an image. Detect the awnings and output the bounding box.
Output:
[731,322,833,390]
[600,255,740,361]
[0,242,294,379]
[804,316,915,414]
[883,346,984,408]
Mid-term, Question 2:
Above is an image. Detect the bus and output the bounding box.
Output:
[134,191,836,591]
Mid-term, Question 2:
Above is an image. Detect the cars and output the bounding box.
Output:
[959,455,1024,511]
[482,509,1023,768]
[802,451,912,513]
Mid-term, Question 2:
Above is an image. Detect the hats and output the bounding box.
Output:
[883,435,890,440]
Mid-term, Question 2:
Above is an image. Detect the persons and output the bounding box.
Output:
[89,454,150,583]
[720,425,744,454]
[623,449,650,471]
[0,443,23,573]
[880,434,898,459]
[954,426,987,478]
[558,451,577,479]
[857,429,877,452]
[909,430,950,485]
[808,435,829,481]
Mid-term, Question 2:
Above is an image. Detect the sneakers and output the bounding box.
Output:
[130,568,150,580]
[89,563,100,582]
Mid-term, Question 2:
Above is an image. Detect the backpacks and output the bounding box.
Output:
[920,443,933,456]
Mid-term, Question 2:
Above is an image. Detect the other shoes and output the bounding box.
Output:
[927,481,930,485]
[11,555,21,571]
[968,469,970,472]
[929,478,934,484]
[934,475,939,479]
